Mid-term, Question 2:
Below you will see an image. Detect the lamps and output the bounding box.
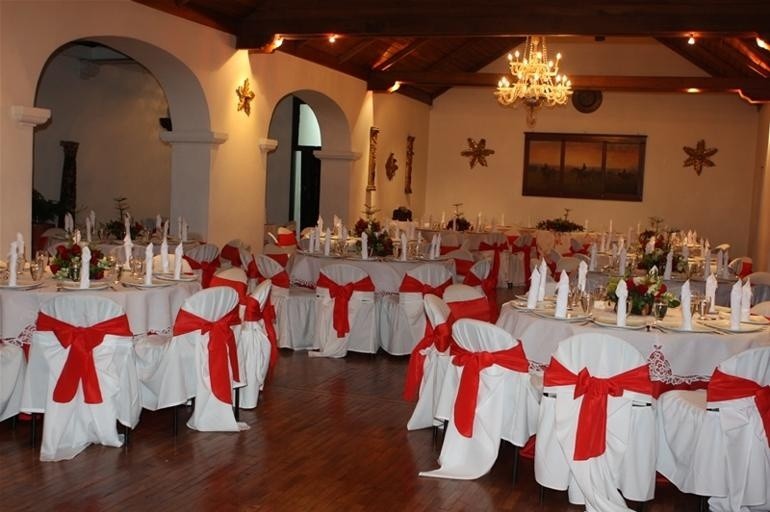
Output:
[489,36,576,125]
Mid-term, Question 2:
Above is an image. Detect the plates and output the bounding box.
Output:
[300,215,770,333]
[58,237,200,245]
[0,265,198,290]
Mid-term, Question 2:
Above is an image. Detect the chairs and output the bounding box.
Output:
[408,213,769,511]
[1,212,408,458]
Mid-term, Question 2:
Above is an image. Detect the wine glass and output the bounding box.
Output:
[141,220,155,241]
[130,253,143,279]
[108,252,122,284]
[30,250,47,280]
[98,222,112,240]
[68,257,80,280]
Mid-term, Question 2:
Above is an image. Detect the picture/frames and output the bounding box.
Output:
[521,126,652,205]
